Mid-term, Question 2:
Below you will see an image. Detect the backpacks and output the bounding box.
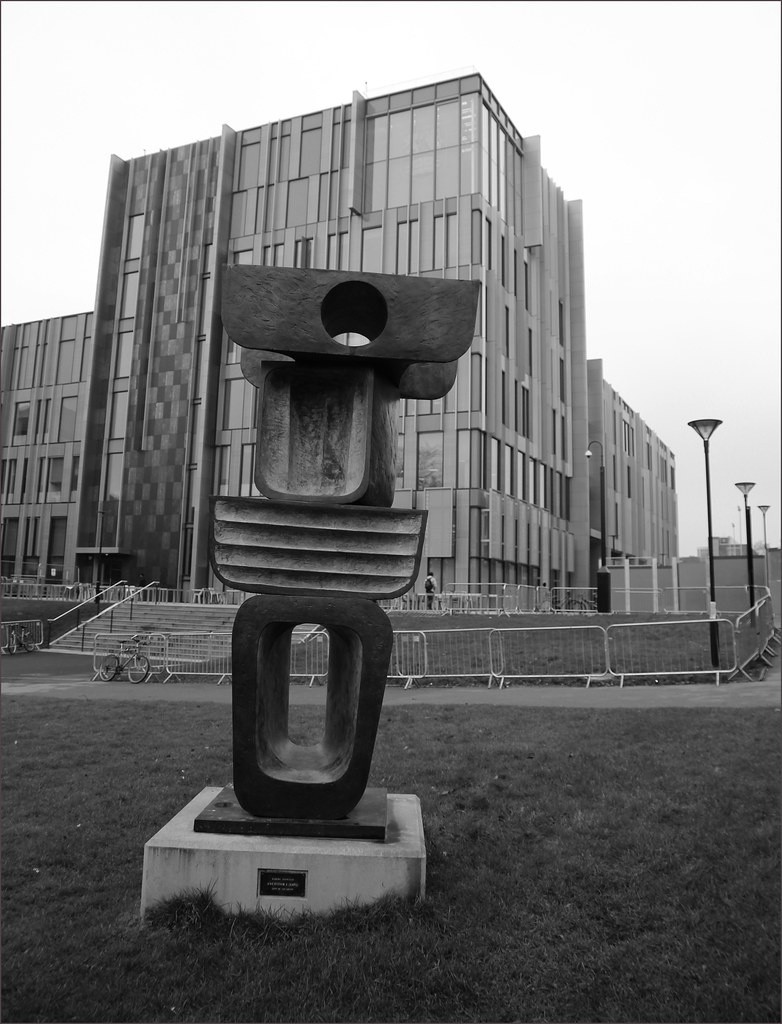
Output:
[425,577,433,590]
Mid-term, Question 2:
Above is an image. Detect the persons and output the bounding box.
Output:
[138,573,147,588]
[425,572,437,610]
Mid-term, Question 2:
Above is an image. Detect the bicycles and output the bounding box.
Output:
[9,624,35,653]
[99,635,150,683]
[539,589,598,615]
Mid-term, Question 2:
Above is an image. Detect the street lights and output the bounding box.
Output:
[688,419,724,671]
[758,505,771,587]
[735,482,756,630]
[585,441,611,614]
